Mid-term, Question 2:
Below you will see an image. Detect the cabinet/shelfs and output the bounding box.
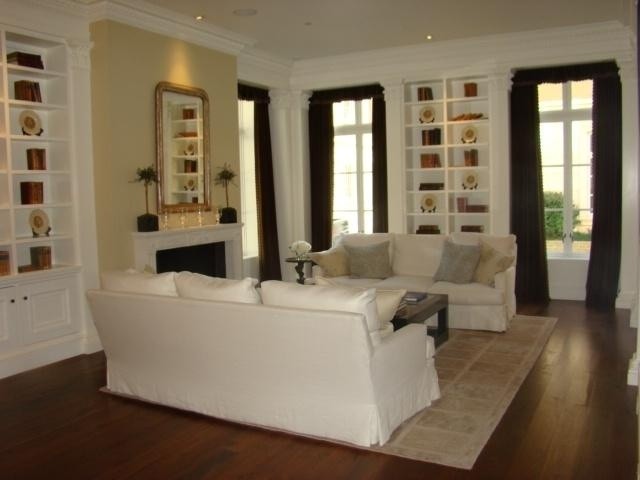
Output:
[1,24,84,288]
[400,74,493,234]
[166,99,203,205]
[0,268,85,381]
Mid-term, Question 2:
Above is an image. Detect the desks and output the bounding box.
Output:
[284,256,313,284]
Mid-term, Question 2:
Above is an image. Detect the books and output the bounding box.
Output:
[18,246,52,273]
[184,160,196,172]
[418,182,445,190]
[464,149,478,166]
[461,225,484,233]
[0,251,11,276]
[403,292,427,302]
[465,82,477,97]
[20,181,43,204]
[417,87,433,101]
[26,148,47,170]
[14,79,41,102]
[420,153,442,169]
[8,52,45,70]
[415,225,440,235]
[467,206,488,211]
[422,128,441,146]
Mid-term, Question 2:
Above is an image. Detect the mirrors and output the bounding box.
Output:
[153,80,213,215]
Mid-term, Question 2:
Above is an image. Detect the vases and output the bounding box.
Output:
[294,250,307,260]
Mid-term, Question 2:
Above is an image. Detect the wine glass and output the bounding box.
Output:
[176,207,189,228]
[160,208,171,231]
[212,207,224,226]
[194,207,207,227]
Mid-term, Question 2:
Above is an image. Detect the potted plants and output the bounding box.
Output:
[125,164,161,232]
[212,161,238,224]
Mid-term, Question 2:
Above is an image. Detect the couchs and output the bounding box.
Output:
[84,267,441,447]
[311,234,517,334]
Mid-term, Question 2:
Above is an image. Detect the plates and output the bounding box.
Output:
[183,142,195,154]
[28,208,50,235]
[420,194,437,211]
[185,177,198,190]
[19,110,42,135]
[419,106,436,123]
[462,170,479,188]
[461,126,479,143]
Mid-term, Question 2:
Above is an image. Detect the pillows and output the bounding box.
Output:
[342,240,394,280]
[471,238,515,289]
[176,271,261,305]
[100,269,178,298]
[432,239,482,284]
[314,275,407,338]
[261,279,382,346]
[303,244,350,278]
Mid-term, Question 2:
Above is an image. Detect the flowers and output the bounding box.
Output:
[290,241,311,254]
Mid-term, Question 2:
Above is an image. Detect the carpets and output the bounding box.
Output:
[97,314,561,472]
